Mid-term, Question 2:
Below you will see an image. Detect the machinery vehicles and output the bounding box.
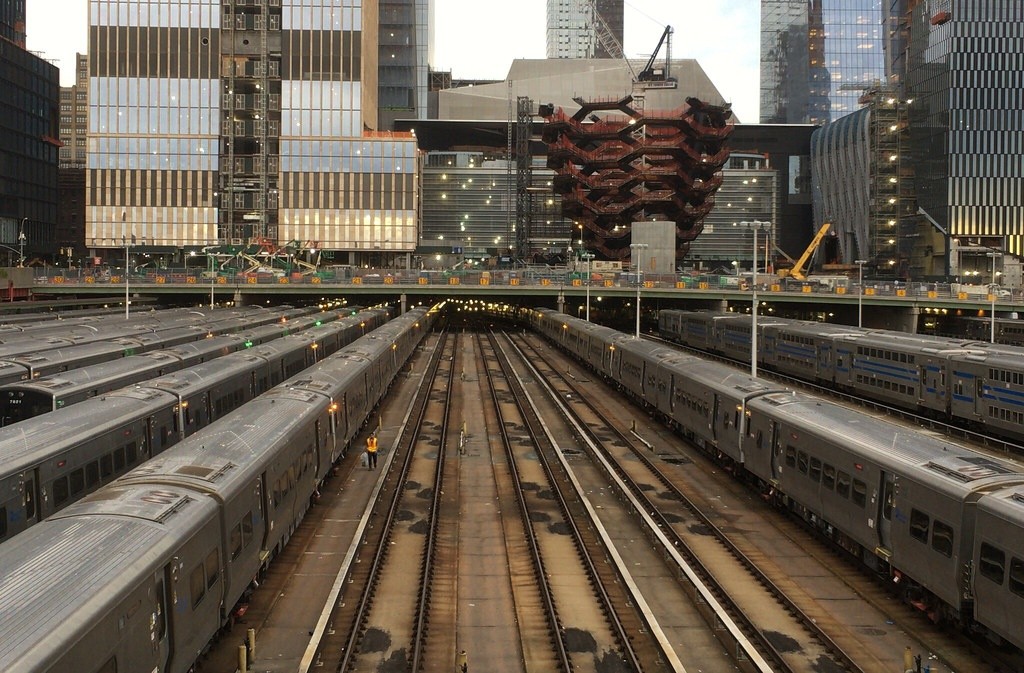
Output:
[772,222,831,292]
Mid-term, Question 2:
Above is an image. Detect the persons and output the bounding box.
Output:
[366,433,378,471]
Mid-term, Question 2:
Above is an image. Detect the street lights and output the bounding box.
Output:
[953,239,962,293]
[853,260,868,327]
[629,244,649,340]
[738,221,772,380]
[579,224,582,256]
[19,218,28,267]
[580,253,596,321]
[986,252,1003,344]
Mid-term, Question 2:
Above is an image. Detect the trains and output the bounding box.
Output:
[660,308,1024,439]
[0,297,434,673]
[531,305,1024,653]
